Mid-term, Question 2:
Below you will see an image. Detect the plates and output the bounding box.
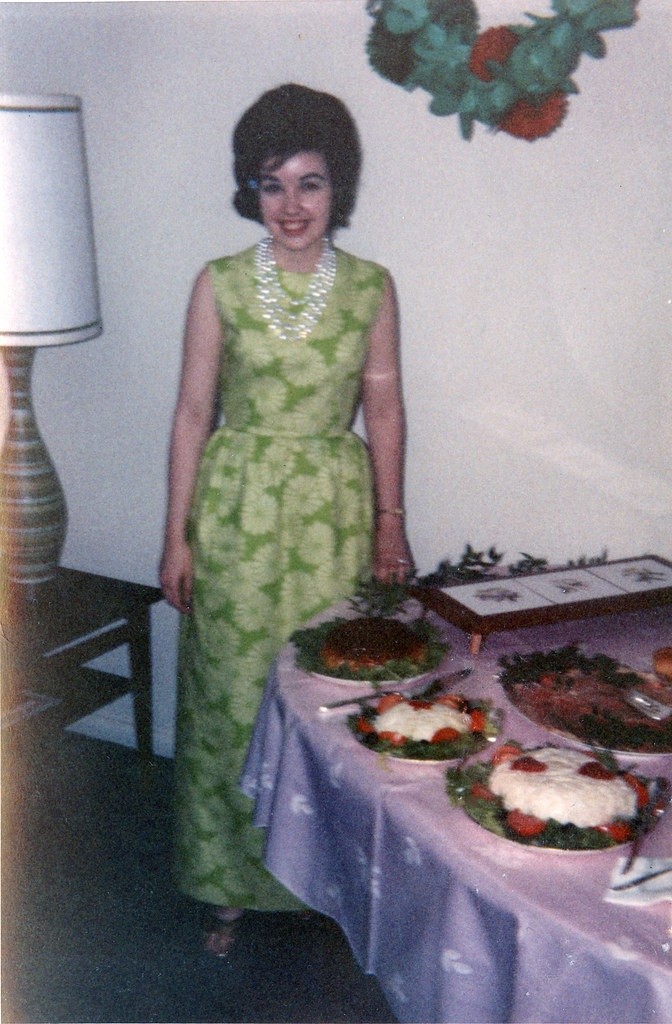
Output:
[502,672,672,759]
[308,669,436,693]
[463,804,638,859]
[356,738,462,766]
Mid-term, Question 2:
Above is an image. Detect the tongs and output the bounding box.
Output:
[613,778,672,891]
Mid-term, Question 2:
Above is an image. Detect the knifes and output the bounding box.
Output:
[414,668,473,700]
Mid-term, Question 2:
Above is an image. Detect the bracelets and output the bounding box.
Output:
[376,504,408,520]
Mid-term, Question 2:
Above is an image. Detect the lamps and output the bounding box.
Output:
[0,93,103,585]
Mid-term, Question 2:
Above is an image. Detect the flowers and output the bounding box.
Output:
[365,0,638,143]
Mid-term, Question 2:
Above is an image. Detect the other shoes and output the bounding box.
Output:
[206,915,242,958]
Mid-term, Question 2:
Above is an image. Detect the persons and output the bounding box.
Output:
[160,84,415,974]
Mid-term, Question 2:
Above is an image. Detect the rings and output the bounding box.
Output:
[397,559,412,566]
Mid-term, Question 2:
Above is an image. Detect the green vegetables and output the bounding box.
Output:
[288,610,672,851]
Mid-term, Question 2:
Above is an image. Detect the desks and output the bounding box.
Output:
[241,583,672,1024]
[2,566,168,817]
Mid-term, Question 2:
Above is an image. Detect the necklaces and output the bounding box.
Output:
[255,237,337,341]
